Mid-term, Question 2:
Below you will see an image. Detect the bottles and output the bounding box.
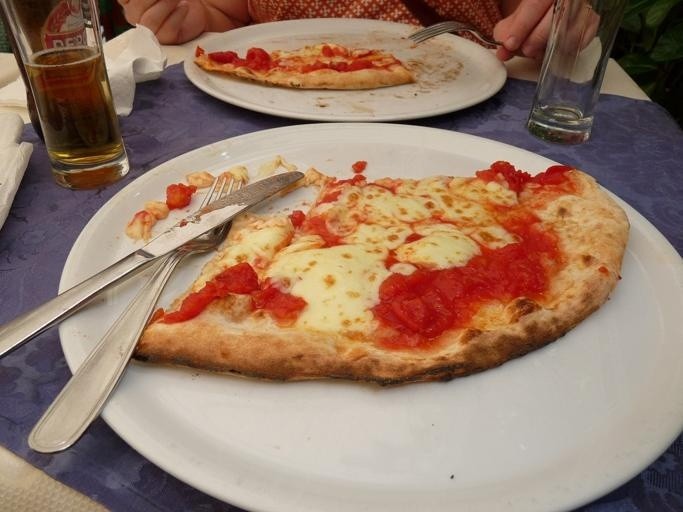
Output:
[0,0,87,144]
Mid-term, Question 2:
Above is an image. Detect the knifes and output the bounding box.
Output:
[0,170,305,357]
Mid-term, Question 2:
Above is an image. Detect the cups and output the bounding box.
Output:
[3,0,130,190]
[526,0,622,144]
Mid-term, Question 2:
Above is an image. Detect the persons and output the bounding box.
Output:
[116,0,606,61]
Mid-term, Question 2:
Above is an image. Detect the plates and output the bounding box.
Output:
[185,19,508,121]
[59,121,682,511]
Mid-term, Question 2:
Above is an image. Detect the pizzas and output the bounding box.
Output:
[193,43,416,90]
[132,160,630,388]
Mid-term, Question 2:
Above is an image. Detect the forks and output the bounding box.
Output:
[27,176,243,456]
[408,19,530,58]
